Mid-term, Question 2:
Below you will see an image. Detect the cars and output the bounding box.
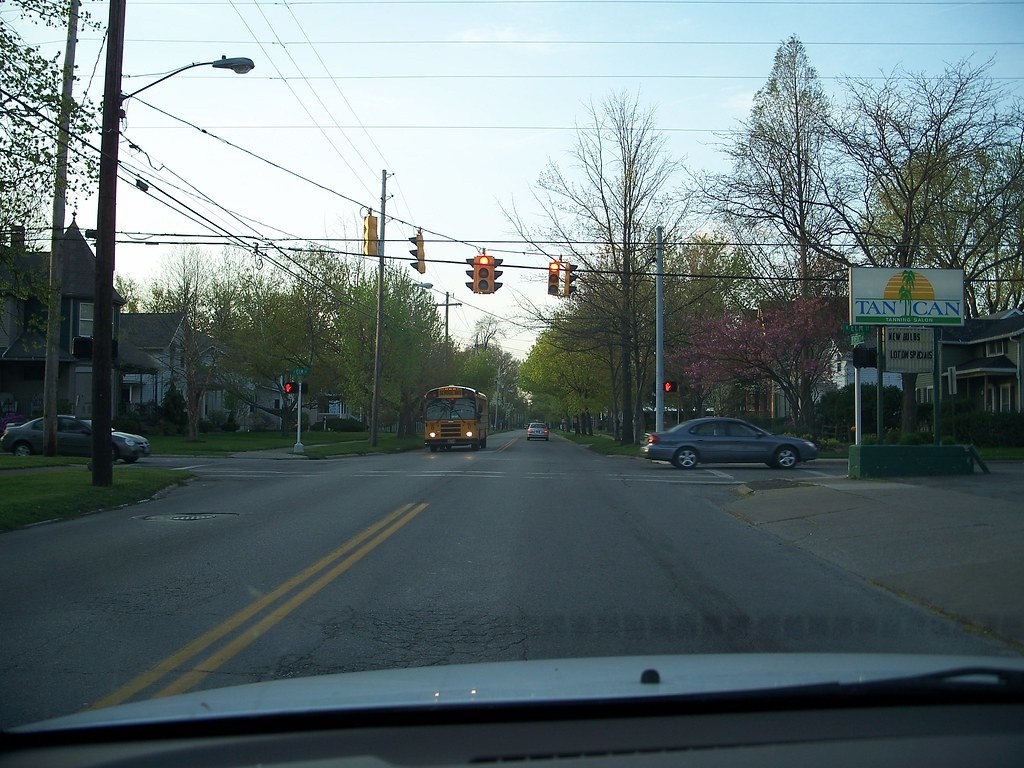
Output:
[0,415,150,463]
[642,416,817,470]
[527,423,549,441]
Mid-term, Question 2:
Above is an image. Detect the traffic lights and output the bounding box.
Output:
[564,264,578,297]
[663,382,676,392]
[466,257,476,292]
[549,263,559,295]
[285,383,297,393]
[409,236,425,274]
[477,256,492,293]
[492,257,503,293]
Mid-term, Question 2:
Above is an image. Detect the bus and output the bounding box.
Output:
[420,385,489,452]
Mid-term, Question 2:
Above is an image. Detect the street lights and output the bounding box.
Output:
[371,284,433,441]
[91,58,255,487]
